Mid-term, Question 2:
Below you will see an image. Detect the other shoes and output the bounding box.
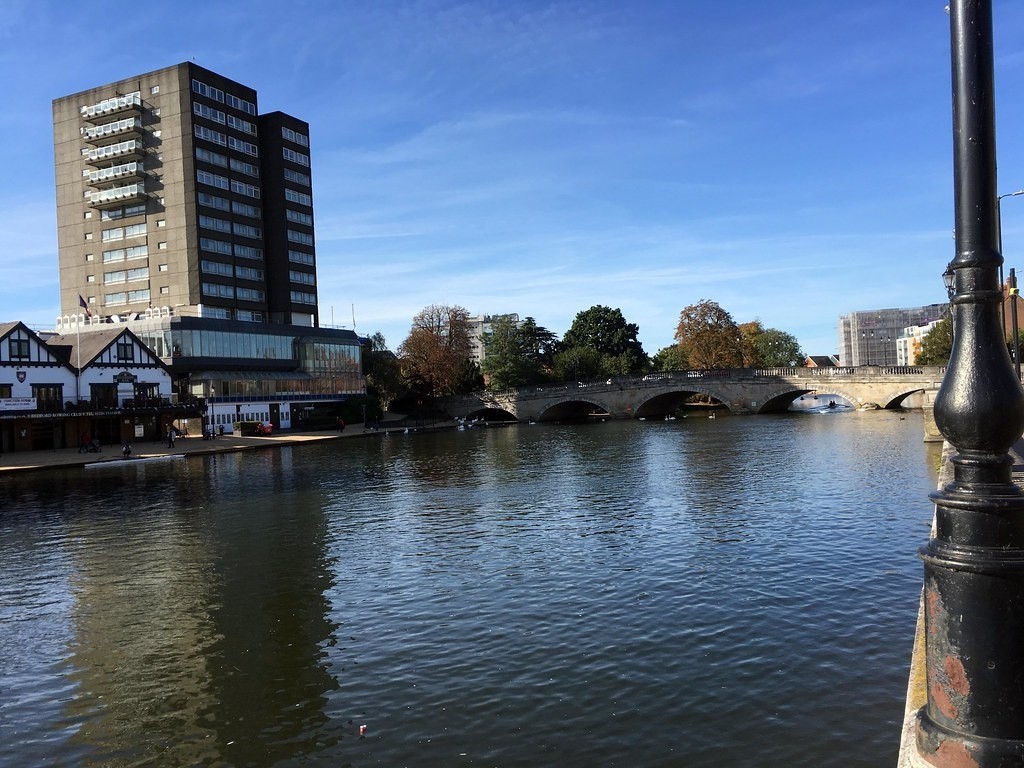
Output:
[173,446,174,448]
[168,447,171,448]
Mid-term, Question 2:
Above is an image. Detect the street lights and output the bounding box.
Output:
[880,335,891,366]
[736,336,747,368]
[210,384,216,439]
[76,333,108,448]
[942,263,956,343]
[769,337,779,367]
[863,329,874,366]
[997,190,1024,340]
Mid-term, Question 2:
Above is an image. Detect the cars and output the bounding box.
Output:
[642,374,666,381]
[688,372,703,377]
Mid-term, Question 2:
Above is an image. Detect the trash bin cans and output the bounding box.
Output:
[216,425,224,436]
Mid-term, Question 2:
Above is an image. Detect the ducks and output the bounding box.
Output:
[453,415,489,432]
[707,413,716,419]
[601,412,689,422]
[899,416,906,421]
[385,427,418,436]
[528,420,536,425]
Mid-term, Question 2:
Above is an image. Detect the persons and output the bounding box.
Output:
[336,416,345,432]
[255,423,263,436]
[80,432,90,453]
[167,428,176,448]
[373,415,380,431]
[123,444,131,456]
[90,439,101,453]
